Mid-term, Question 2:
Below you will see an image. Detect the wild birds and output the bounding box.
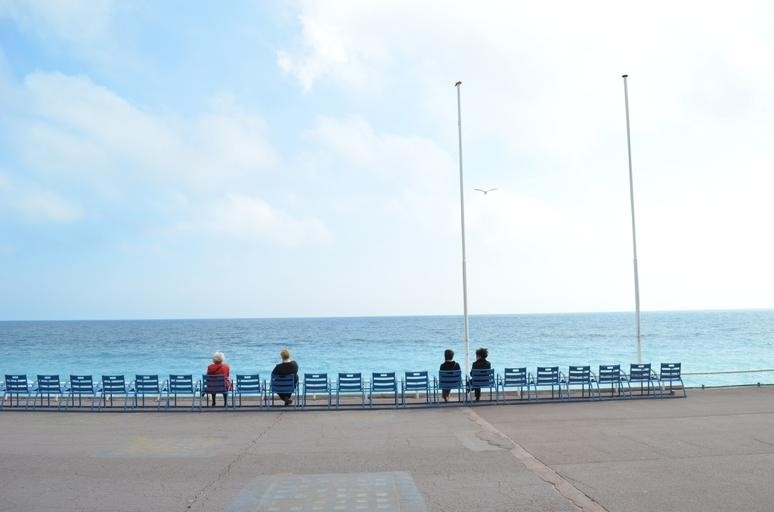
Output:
[475,189,496,194]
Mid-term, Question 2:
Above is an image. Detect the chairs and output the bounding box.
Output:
[496,367,529,401]
[0,373,36,411]
[33,373,68,411]
[399,370,433,407]
[166,372,202,412]
[432,369,468,406]
[528,366,564,402]
[369,371,400,408]
[64,372,104,412]
[334,371,367,409]
[465,368,499,406]
[97,374,136,411]
[267,373,301,409]
[651,362,686,399]
[559,365,596,400]
[199,373,234,410]
[590,364,627,399]
[301,372,333,409]
[234,372,267,408]
[620,363,658,399]
[131,372,171,411]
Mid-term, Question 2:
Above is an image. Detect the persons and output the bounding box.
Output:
[467,348,491,400]
[272,350,298,405]
[207,353,232,406]
[440,350,461,402]
